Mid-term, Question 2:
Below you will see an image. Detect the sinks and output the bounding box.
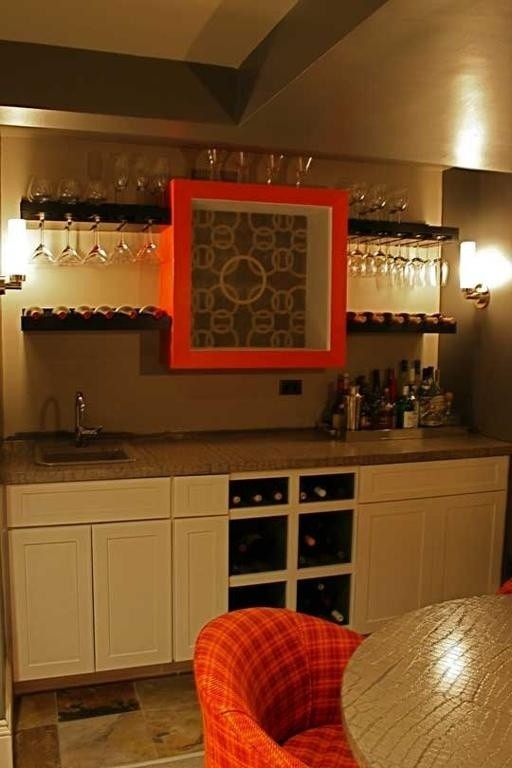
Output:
[33,442,137,466]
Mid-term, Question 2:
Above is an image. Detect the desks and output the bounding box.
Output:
[339,596,512,765]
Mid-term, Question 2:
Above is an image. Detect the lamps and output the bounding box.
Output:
[457,236,490,309]
[0,213,25,294]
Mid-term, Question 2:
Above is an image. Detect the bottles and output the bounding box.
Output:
[231,478,349,625]
[23,304,163,320]
[327,360,455,430]
[347,311,455,325]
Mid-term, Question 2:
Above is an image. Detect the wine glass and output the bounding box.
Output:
[347,233,450,287]
[348,181,410,224]
[30,212,164,265]
[202,148,314,187]
[24,151,170,206]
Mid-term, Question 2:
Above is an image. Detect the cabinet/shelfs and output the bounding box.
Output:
[19,199,171,335]
[229,458,358,634]
[9,473,169,693]
[172,472,232,673]
[159,177,350,370]
[348,219,458,335]
[353,450,512,641]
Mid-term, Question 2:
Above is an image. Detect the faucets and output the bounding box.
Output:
[74,391,103,446]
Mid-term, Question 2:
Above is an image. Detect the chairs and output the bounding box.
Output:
[191,607,366,767]
